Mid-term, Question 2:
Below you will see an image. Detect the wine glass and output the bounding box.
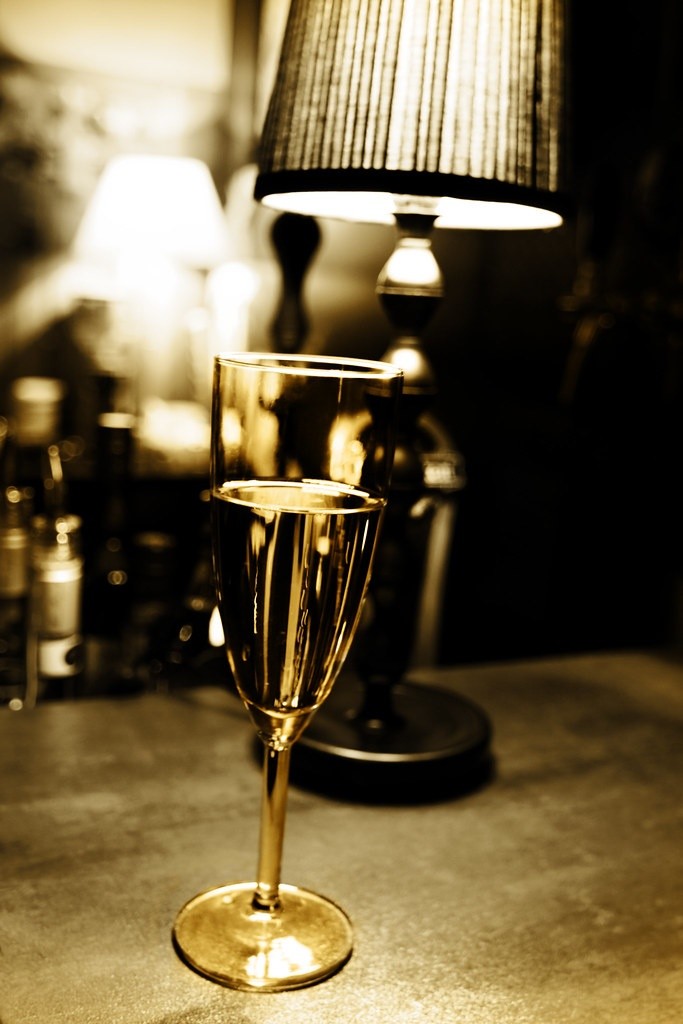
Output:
[173,355,406,994]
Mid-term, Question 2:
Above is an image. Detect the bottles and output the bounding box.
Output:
[0,373,143,682]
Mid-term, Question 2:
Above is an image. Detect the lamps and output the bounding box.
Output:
[251,0,567,803]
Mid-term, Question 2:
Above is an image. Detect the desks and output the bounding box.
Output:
[0,650,682,1024]
